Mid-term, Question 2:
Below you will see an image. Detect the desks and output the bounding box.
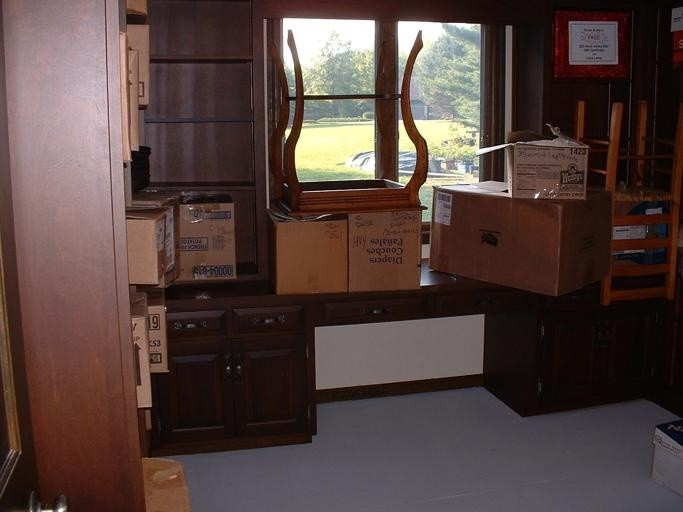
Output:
[263,24,431,208]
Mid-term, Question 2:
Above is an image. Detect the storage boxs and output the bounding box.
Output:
[269,209,424,296]
[652,419,683,495]
[429,121,610,297]
[125,286,168,408]
[126,1,149,162]
[125,190,235,285]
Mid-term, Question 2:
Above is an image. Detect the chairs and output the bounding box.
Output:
[572,96,681,307]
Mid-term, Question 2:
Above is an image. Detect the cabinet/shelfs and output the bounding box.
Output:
[148,259,322,458]
[141,0,272,284]
[482,280,653,419]
[2,0,163,512]
